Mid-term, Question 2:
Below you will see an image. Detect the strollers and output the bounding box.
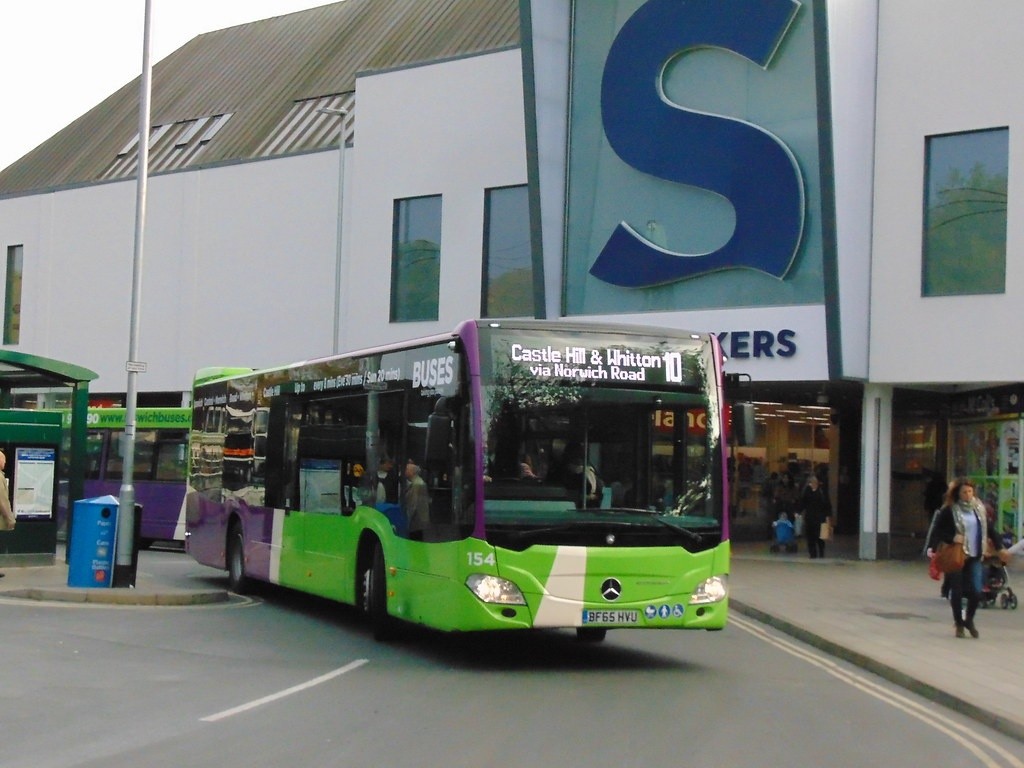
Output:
[978,531,1017,609]
[770,500,798,552]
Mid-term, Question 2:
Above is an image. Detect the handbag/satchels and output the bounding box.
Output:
[819,518,833,545]
[936,539,965,572]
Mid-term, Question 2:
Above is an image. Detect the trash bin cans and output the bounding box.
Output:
[68,495,143,589]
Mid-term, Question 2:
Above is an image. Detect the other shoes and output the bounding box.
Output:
[955,619,979,639]
[809,553,825,559]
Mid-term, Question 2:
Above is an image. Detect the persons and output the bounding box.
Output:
[0,451,18,578]
[791,474,834,558]
[404,461,432,539]
[775,510,796,533]
[463,424,609,507]
[922,481,1024,638]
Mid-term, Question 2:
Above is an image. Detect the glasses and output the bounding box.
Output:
[809,482,817,486]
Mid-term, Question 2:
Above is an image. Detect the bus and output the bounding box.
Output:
[35,409,193,549]
[185,317,755,642]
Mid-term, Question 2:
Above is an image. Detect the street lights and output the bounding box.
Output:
[319,108,346,352]
